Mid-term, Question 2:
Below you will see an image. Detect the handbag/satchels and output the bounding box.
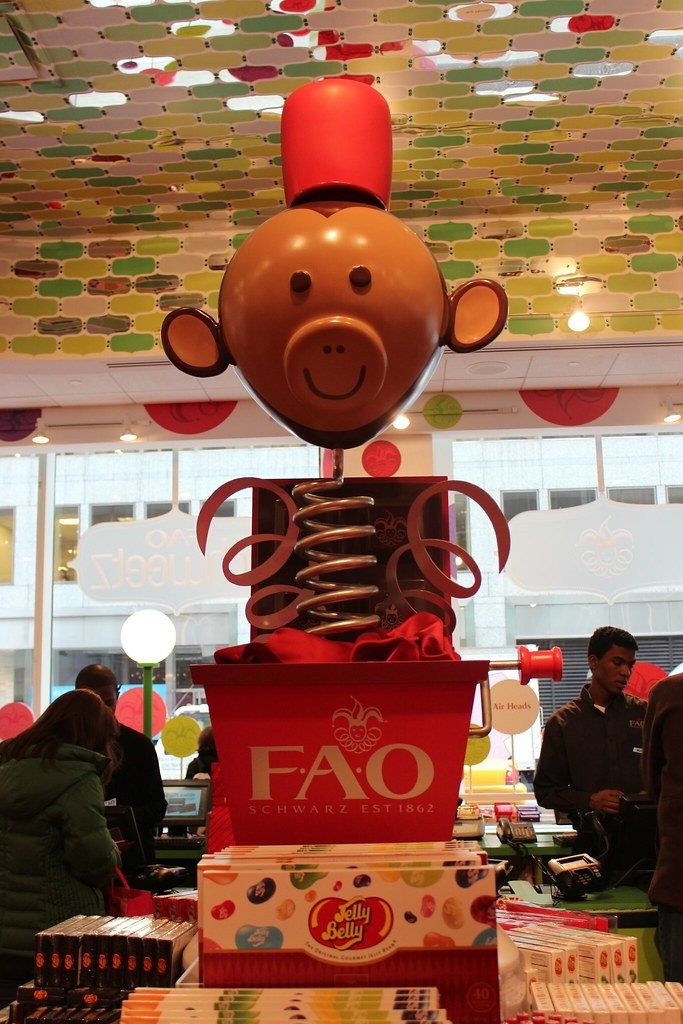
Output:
[102,865,156,917]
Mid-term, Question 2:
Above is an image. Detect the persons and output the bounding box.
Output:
[532,627,658,889]
[639,670,683,984]
[71,662,169,878]
[182,724,219,780]
[0,688,125,1015]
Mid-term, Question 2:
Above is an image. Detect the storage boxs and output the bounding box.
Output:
[0,891,683,1024]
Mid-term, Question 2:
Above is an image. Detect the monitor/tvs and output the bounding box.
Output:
[611,794,658,870]
[161,779,210,837]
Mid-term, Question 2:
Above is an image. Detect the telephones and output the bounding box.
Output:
[497,816,537,844]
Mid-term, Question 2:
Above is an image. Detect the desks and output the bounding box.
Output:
[87,822,665,985]
